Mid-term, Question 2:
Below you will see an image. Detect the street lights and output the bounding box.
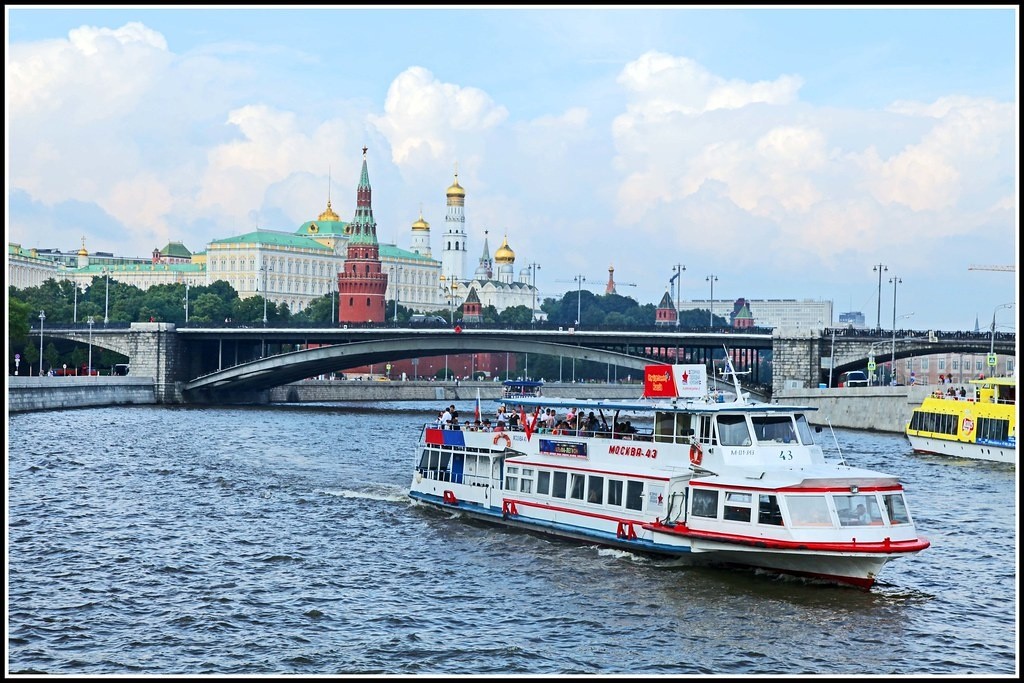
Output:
[447,273,458,326]
[38,310,46,376]
[527,261,541,330]
[705,273,718,327]
[990,305,1012,377]
[182,277,193,323]
[889,275,903,335]
[873,262,889,331]
[71,277,82,322]
[260,265,272,328]
[101,267,114,323]
[672,263,686,327]
[573,274,586,324]
[86,316,95,375]
[390,263,403,322]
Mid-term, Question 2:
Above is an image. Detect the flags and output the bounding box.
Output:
[475,393,479,420]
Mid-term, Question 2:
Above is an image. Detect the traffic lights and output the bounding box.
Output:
[892,367,897,377]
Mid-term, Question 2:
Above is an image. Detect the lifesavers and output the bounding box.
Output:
[493,433,512,449]
[690,442,701,465]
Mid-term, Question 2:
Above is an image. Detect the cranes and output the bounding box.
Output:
[554,279,637,288]
[669,273,679,302]
[968,262,1015,271]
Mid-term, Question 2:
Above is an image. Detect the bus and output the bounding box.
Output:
[838,371,868,389]
[115,363,130,375]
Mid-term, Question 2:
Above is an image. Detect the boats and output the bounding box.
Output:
[407,342,931,592]
[906,376,1015,465]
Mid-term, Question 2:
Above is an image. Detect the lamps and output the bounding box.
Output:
[850,485,858,493]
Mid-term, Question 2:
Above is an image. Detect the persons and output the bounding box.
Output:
[464,418,493,432]
[940,373,953,383]
[873,378,879,385]
[39,370,44,377]
[852,503,871,524]
[990,396,1009,404]
[931,388,944,398]
[947,386,966,397]
[494,406,637,440]
[435,405,458,430]
[47,368,53,377]
